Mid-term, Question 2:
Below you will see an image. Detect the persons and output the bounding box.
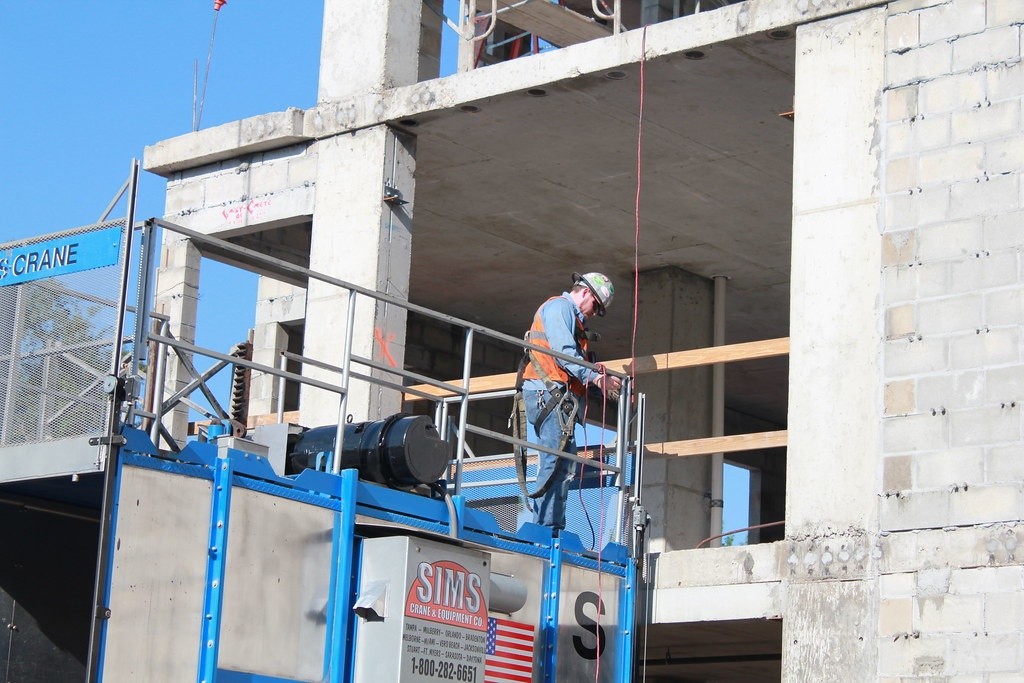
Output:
[509,270,623,549]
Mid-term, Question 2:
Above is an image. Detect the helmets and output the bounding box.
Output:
[573,272,614,316]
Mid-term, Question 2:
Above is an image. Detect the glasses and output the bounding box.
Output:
[591,293,604,317]
